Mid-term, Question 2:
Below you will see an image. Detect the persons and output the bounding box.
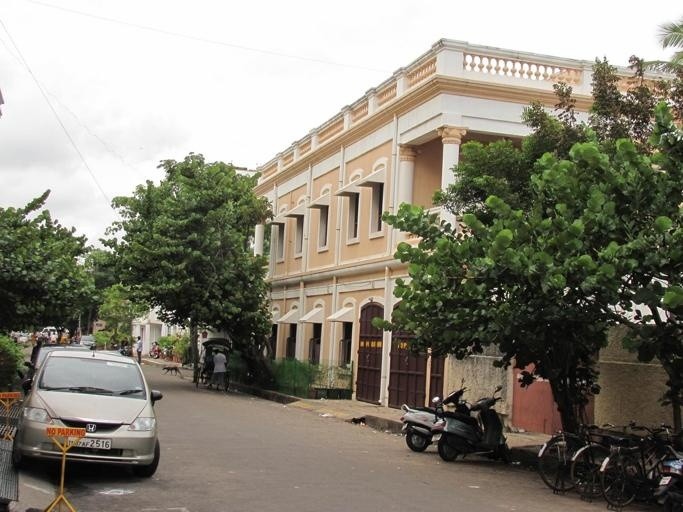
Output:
[206,349,227,389]
[121,336,128,355]
[136,336,142,363]
[149,341,159,358]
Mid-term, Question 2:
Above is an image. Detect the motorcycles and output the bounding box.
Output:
[653,457,683,512]
[400,378,483,452]
[430,384,513,464]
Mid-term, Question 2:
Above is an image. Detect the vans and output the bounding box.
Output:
[40,326,70,338]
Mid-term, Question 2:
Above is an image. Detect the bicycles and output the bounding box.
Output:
[537,420,679,512]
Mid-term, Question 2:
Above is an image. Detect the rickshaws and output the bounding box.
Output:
[200,341,232,393]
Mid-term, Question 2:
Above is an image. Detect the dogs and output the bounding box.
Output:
[162,366,181,377]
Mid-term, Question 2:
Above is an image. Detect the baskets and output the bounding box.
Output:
[656,434,682,454]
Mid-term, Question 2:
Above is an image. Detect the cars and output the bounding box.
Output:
[79,336,97,349]
[9,344,163,477]
